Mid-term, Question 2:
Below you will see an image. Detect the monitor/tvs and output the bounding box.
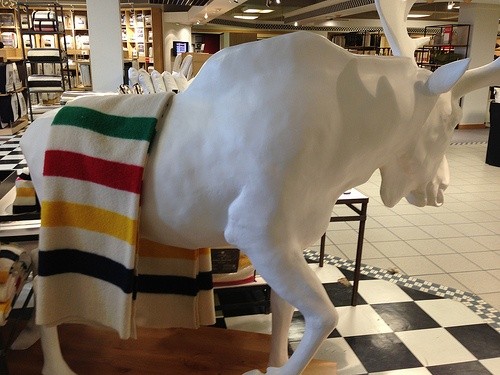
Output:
[173,41,188,57]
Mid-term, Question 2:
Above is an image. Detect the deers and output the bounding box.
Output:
[19,1,500,375]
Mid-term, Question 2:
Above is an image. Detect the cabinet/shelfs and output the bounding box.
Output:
[328,23,393,58]
[414,24,471,129]
[0,0,164,136]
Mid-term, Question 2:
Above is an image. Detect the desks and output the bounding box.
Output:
[319,187,369,306]
